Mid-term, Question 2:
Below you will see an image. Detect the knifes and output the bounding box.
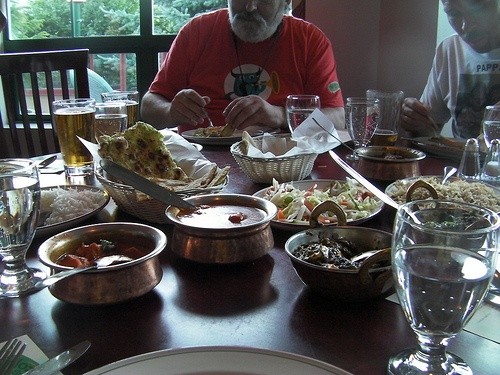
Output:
[21,340,91,375]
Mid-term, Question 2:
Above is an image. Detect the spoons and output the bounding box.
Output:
[38,156,57,169]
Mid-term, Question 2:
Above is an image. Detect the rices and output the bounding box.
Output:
[33,186,108,228]
[391,176,500,214]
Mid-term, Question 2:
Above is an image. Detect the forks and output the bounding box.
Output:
[0,336,27,375]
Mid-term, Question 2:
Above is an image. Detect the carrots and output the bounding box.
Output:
[278,209,285,220]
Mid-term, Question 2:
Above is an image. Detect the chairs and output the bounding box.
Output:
[0,49,90,159]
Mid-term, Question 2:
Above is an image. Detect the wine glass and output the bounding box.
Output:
[0,160,48,300]
[387,200,499,375]
[346,97,381,160]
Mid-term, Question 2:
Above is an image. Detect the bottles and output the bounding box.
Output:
[456,138,500,187]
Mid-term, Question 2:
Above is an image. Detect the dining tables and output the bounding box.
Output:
[0,136,500,375]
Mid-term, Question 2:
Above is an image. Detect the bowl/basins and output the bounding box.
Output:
[38,181,500,302]
[352,145,426,181]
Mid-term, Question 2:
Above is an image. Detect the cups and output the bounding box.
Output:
[366,88,404,146]
[51,91,140,180]
[483,106,500,156]
[286,94,321,134]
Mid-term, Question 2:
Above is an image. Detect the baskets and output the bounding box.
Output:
[229,133,320,184]
[95,165,230,223]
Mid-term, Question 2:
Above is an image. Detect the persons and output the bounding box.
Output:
[401,1,500,156]
[138,0,346,135]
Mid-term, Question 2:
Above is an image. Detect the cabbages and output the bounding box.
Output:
[262,178,380,224]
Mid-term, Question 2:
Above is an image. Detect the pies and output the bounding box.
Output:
[96,120,191,183]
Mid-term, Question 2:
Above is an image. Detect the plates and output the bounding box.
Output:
[412,136,468,156]
[82,345,354,375]
[252,174,500,230]
[182,126,282,144]
[36,182,109,233]
